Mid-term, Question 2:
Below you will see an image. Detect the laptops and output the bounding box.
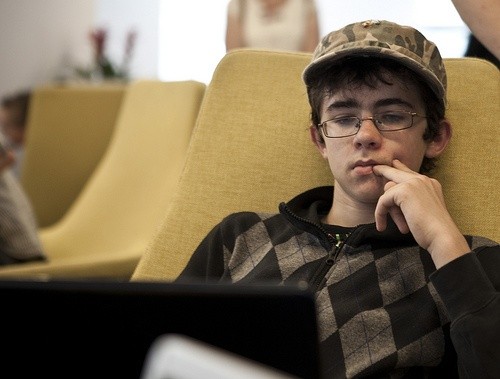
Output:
[0,279,324,379]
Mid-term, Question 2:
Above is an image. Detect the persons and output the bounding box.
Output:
[225,0,318,54]
[0,90,46,264]
[171,19,500,379]
[452,0,500,67]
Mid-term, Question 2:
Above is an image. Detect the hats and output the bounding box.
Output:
[301,19,448,105]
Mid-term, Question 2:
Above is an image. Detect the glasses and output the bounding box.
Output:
[318,111,428,138]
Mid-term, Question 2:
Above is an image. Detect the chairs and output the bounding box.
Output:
[0,47,500,280]
